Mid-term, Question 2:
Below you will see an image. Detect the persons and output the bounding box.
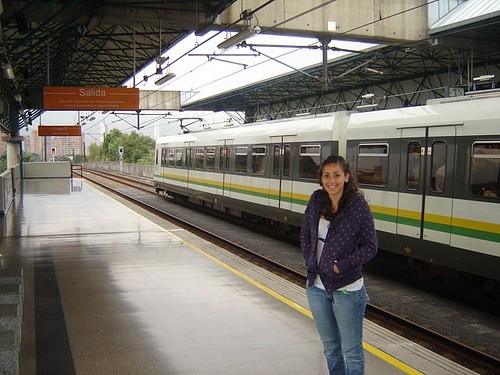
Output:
[300,155,378,375]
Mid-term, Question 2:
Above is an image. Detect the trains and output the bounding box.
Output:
[154,88,500,310]
